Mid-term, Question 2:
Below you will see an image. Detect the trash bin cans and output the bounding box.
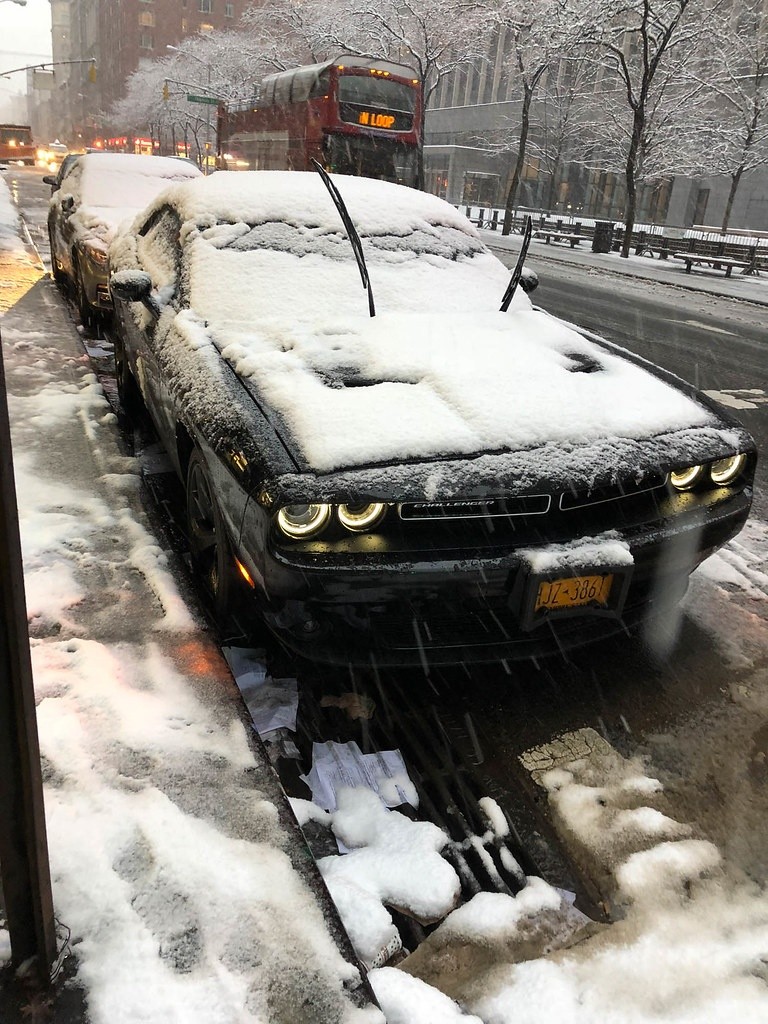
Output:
[592,221,616,253]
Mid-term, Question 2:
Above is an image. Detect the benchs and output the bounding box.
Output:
[673,253,751,278]
[537,230,587,248]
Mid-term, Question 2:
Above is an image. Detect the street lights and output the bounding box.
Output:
[160,43,213,175]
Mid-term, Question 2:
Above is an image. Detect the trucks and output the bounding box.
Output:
[0,122,37,166]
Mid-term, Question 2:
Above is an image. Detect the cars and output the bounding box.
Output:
[48,153,210,323]
[42,152,89,202]
[106,158,759,675]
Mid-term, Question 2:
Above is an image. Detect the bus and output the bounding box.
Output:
[214,53,426,192]
